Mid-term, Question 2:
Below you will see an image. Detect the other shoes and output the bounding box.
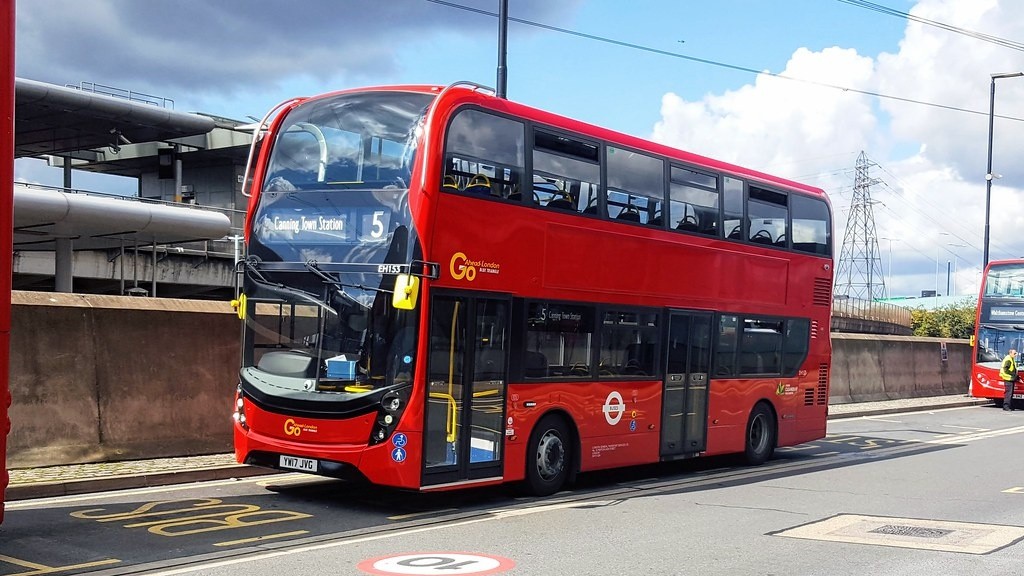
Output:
[1003,406,1010,411]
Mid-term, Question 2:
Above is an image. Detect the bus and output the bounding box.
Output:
[229,80,832,497]
[969,259,1024,408]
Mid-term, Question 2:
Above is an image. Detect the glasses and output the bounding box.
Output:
[1012,353,1017,355]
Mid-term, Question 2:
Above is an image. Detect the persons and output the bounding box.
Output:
[999,348,1023,412]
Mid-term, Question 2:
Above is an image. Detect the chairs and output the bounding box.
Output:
[569,359,732,378]
[443,172,789,254]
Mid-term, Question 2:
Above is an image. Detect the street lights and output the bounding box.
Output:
[948,243,967,298]
[983,72,1024,276]
[935,232,948,297]
[882,238,899,300]
